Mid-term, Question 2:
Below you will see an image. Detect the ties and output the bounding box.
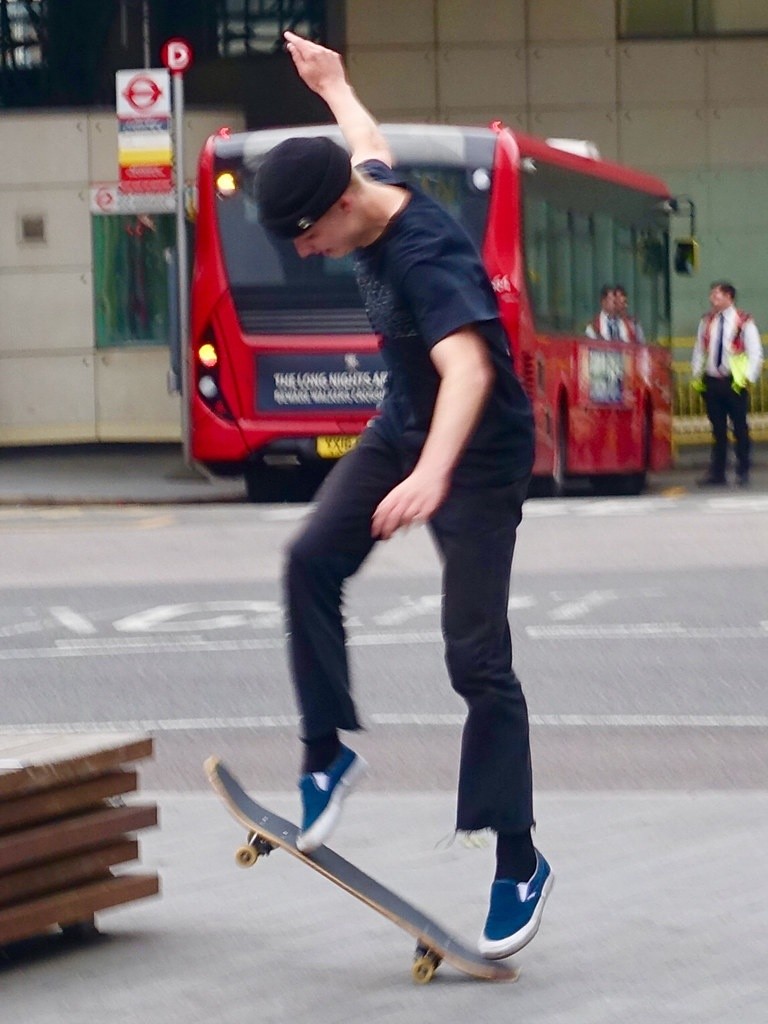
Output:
[609,319,620,340]
[715,313,723,367]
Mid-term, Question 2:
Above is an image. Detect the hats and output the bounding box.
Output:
[253,137,351,239]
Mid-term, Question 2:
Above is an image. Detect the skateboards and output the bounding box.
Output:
[202,753,521,986]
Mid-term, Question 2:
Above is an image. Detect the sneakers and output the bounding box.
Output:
[478,846,554,960]
[297,742,368,852]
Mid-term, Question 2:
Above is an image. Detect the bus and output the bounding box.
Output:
[188,119,698,500]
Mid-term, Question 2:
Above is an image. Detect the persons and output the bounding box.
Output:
[584,285,651,470]
[253,32,554,961]
[691,280,762,488]
